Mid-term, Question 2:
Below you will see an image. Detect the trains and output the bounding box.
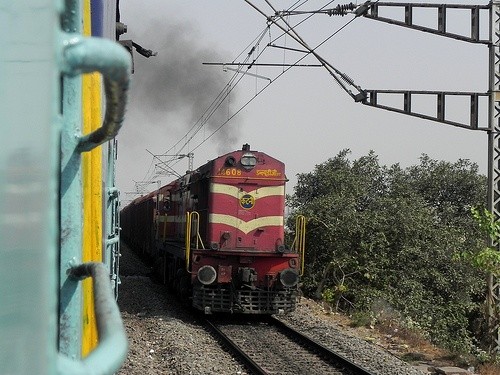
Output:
[119,141,305,319]
[0,0,156,375]
